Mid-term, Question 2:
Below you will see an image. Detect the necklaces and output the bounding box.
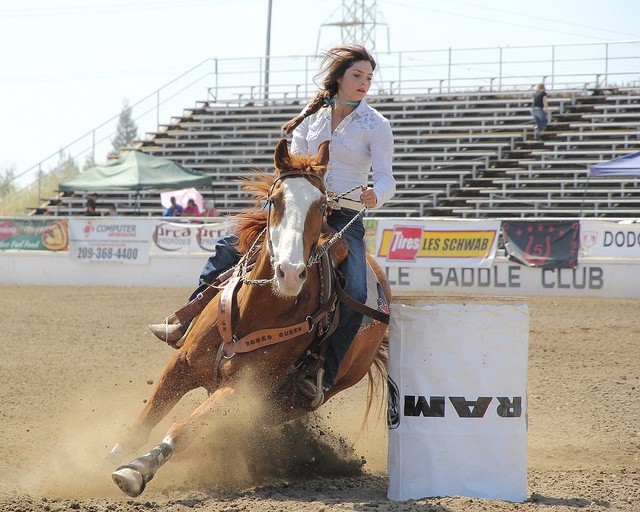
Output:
[322,97,360,109]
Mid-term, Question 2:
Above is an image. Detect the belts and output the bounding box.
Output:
[338,199,366,212]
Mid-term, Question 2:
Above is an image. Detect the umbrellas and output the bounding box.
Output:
[159,188,204,212]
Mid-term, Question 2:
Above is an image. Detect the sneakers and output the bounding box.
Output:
[298,375,331,398]
[148,321,192,344]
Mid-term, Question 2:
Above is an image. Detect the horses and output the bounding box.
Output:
[92,139,392,498]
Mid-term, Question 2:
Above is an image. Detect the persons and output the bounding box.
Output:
[146,43,399,401]
[83,194,101,216]
[530,83,552,140]
[181,199,200,218]
[108,204,123,216]
[163,195,184,216]
